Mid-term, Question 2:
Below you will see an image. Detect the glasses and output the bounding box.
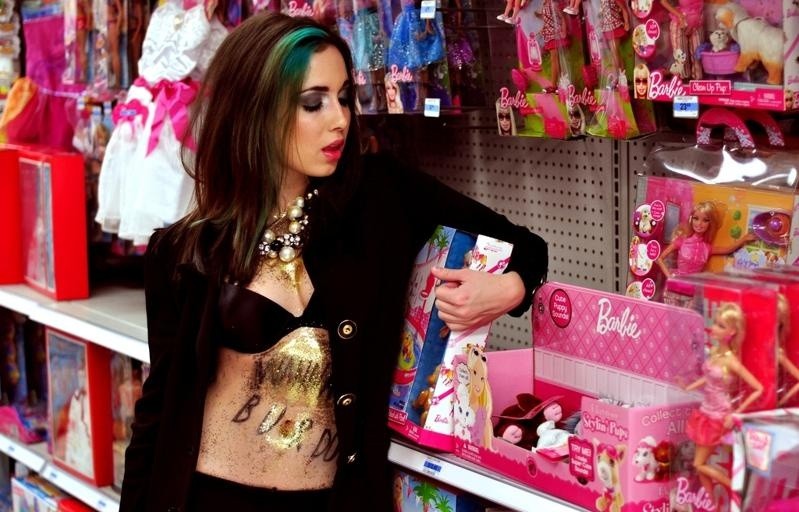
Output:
[498,112,511,120]
[633,77,649,85]
[568,110,580,120]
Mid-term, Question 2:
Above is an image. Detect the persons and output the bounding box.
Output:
[0,0,799,512]
[118,12,548,512]
[337,0,799,507]
[0,0,337,477]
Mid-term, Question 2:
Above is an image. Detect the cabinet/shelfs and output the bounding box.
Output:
[0,0,799,512]
